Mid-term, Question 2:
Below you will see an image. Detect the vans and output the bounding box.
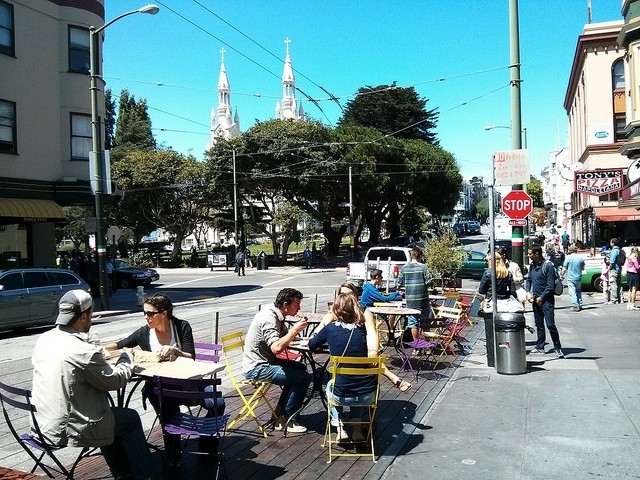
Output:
[0,268,92,333]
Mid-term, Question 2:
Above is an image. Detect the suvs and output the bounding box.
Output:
[453,223,465,236]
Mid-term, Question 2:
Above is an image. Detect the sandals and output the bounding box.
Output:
[394,378,412,393]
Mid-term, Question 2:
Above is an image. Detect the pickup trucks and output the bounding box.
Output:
[346,247,412,296]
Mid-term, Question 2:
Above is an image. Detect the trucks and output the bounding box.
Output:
[467,221,480,227]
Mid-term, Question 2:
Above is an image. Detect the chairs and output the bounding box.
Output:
[219,332,281,437]
[322,355,386,465]
[368,307,411,378]
[396,317,444,382]
[445,291,477,326]
[153,375,229,480]
[1,382,86,480]
[145,339,225,464]
[415,307,464,368]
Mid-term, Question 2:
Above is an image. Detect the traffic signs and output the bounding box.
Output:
[98,244,107,252]
[493,149,530,187]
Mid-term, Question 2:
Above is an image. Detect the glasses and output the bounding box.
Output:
[380,276,383,279]
[143,310,162,318]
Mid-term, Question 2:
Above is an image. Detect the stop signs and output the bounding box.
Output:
[503,191,533,220]
[509,220,527,226]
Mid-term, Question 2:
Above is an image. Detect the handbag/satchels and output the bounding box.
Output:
[479,297,494,313]
[234,264,238,273]
[510,262,527,303]
[326,324,355,373]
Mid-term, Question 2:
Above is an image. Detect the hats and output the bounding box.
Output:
[55,289,94,328]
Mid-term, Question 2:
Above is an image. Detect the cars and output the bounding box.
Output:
[581,247,627,293]
[444,249,488,280]
[111,259,159,288]
[467,225,480,235]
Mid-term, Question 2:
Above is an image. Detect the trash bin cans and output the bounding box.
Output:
[478,296,526,367]
[260,253,269,270]
[494,312,526,375]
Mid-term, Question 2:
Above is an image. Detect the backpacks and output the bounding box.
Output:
[614,247,626,267]
[543,260,563,296]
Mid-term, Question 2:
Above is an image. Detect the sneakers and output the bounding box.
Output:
[555,348,564,358]
[627,304,631,310]
[568,307,581,312]
[631,305,640,311]
[336,431,349,443]
[352,433,365,441]
[529,348,545,355]
[274,421,308,433]
[608,301,618,305]
[619,301,624,304]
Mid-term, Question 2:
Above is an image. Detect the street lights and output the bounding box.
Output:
[88,4,160,311]
[487,125,528,149]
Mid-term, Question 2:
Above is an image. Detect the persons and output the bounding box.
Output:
[361,269,402,339]
[56,250,96,271]
[312,242,318,266]
[395,247,436,356]
[106,256,115,298]
[607,238,624,305]
[104,291,225,467]
[495,247,524,302]
[544,223,570,272]
[245,249,254,268]
[538,232,545,246]
[600,257,609,305]
[303,246,312,269]
[525,244,564,358]
[308,293,380,448]
[235,249,245,276]
[242,288,312,433]
[560,245,586,312]
[309,282,413,393]
[30,289,151,480]
[626,247,640,311]
[478,252,524,301]
